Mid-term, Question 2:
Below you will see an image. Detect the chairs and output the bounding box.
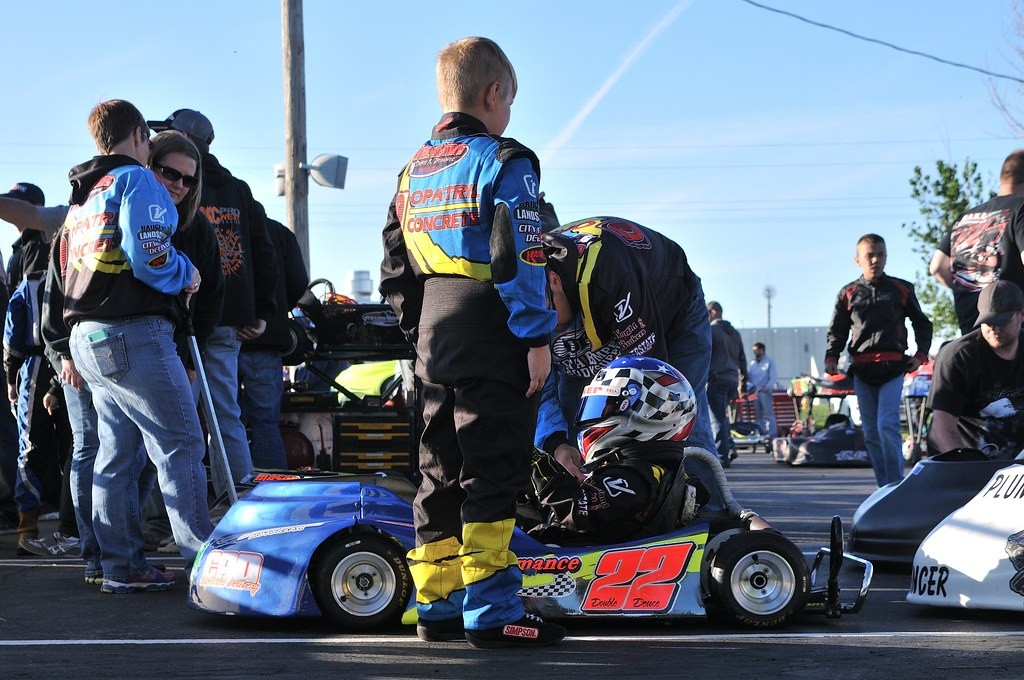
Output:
[629,456,689,540]
[824,414,854,430]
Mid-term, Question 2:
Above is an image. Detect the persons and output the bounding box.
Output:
[0,99,309,592]
[378,36,565,648]
[516,217,778,550]
[930,150,1024,335]
[929,280,1024,457]
[824,234,933,488]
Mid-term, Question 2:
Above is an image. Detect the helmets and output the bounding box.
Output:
[573,356,698,463]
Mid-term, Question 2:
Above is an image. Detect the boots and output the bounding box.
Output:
[16,510,39,556]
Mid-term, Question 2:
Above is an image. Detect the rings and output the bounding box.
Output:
[195,284,198,287]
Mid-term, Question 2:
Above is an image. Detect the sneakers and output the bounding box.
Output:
[416,616,465,641]
[85,565,166,584]
[464,612,566,649]
[16,532,87,560]
[100,566,176,593]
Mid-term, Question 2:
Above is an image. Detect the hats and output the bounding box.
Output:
[0,183,44,207]
[147,108,215,144]
[973,279,1024,327]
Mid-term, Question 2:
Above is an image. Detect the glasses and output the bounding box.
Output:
[547,282,557,310]
[133,132,153,151]
[155,162,199,188]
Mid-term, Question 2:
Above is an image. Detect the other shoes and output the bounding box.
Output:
[156,536,180,552]
[38,506,59,522]
[139,524,170,551]
